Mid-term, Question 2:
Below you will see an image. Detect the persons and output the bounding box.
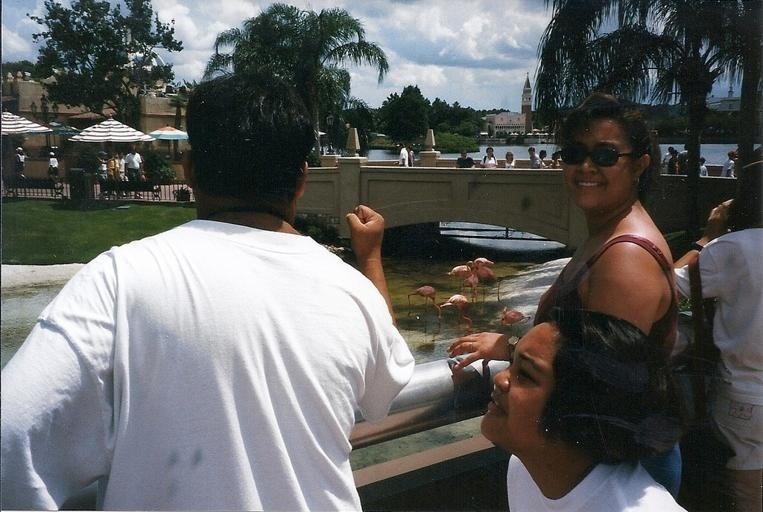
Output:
[672,162,763,512]
[662,147,737,177]
[481,310,702,512]
[47,151,59,186]
[0,68,416,512]
[96,146,145,198]
[15,146,29,180]
[446,92,682,499]
[456,147,563,169]
[393,142,414,167]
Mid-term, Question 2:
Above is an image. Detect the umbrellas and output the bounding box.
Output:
[1,112,53,136]
[149,124,189,153]
[67,114,157,152]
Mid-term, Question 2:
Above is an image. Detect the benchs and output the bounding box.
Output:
[4,176,65,200]
[99,179,160,202]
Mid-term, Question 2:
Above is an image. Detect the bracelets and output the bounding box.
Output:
[688,242,703,252]
[508,335,521,364]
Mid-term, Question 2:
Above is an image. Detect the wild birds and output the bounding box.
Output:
[498,304,531,338]
[438,294,470,324]
[447,258,497,303]
[407,285,437,307]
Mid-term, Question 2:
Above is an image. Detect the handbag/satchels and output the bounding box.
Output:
[661,309,737,474]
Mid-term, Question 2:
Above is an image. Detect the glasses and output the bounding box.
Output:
[560,141,633,167]
